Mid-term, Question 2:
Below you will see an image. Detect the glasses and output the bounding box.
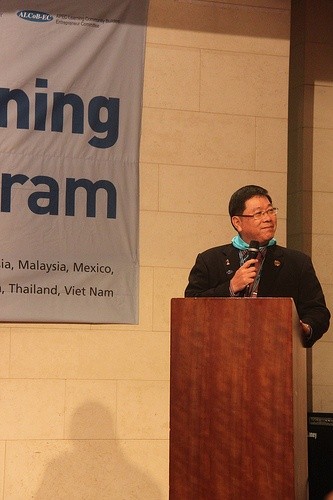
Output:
[237,208,279,220]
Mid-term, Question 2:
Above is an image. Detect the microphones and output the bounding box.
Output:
[242,240,259,297]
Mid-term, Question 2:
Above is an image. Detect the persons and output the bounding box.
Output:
[185,185,330,348]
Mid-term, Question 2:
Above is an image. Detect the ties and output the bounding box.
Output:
[242,247,267,297]
